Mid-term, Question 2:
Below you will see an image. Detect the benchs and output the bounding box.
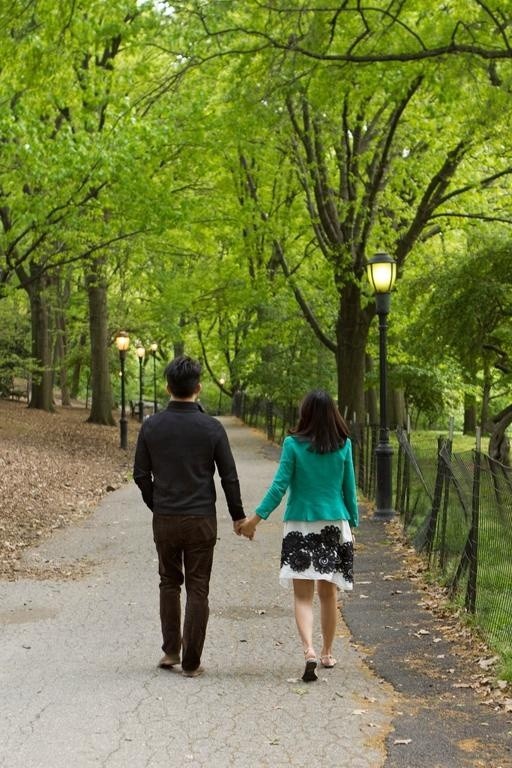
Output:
[130,399,139,417]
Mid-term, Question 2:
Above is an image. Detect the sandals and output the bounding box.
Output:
[320,655,336,668]
[302,649,318,682]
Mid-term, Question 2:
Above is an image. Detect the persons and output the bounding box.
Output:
[132,355,256,679]
[237,389,359,682]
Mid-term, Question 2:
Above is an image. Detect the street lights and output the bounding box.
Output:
[363,248,403,520]
[114,331,159,452]
[215,377,225,416]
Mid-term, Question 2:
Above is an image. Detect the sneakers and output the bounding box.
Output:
[160,653,180,665]
[182,665,204,677]
